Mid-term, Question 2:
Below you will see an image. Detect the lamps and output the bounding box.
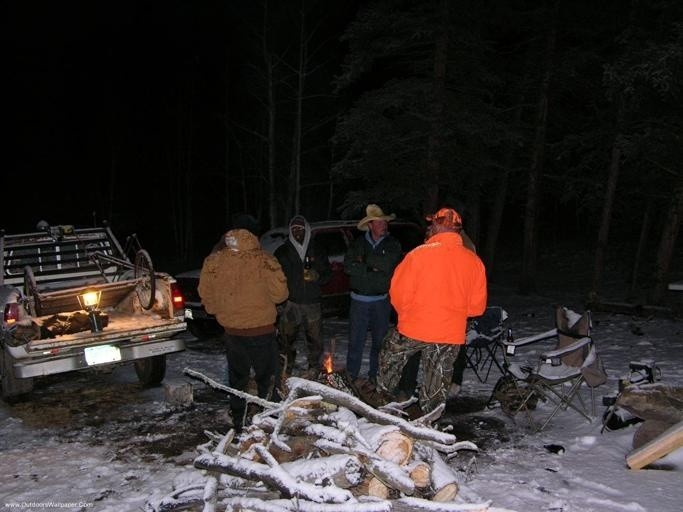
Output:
[75,286,103,334]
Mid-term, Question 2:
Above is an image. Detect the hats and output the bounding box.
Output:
[357,204,396,231]
[290,218,304,231]
[426,207,462,229]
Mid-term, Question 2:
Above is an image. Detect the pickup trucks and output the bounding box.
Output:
[0,216,188,398]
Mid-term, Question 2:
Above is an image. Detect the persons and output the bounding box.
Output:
[344,205,401,381]
[197,214,289,431]
[277,214,332,377]
[400,231,474,397]
[373,208,489,415]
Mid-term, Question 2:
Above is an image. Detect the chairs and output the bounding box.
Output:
[457,303,511,384]
[484,307,604,434]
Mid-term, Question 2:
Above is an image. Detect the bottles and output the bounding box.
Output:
[506,328,513,355]
[544,444,564,454]
[304,256,311,280]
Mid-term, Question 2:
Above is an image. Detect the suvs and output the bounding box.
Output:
[174,219,428,340]
